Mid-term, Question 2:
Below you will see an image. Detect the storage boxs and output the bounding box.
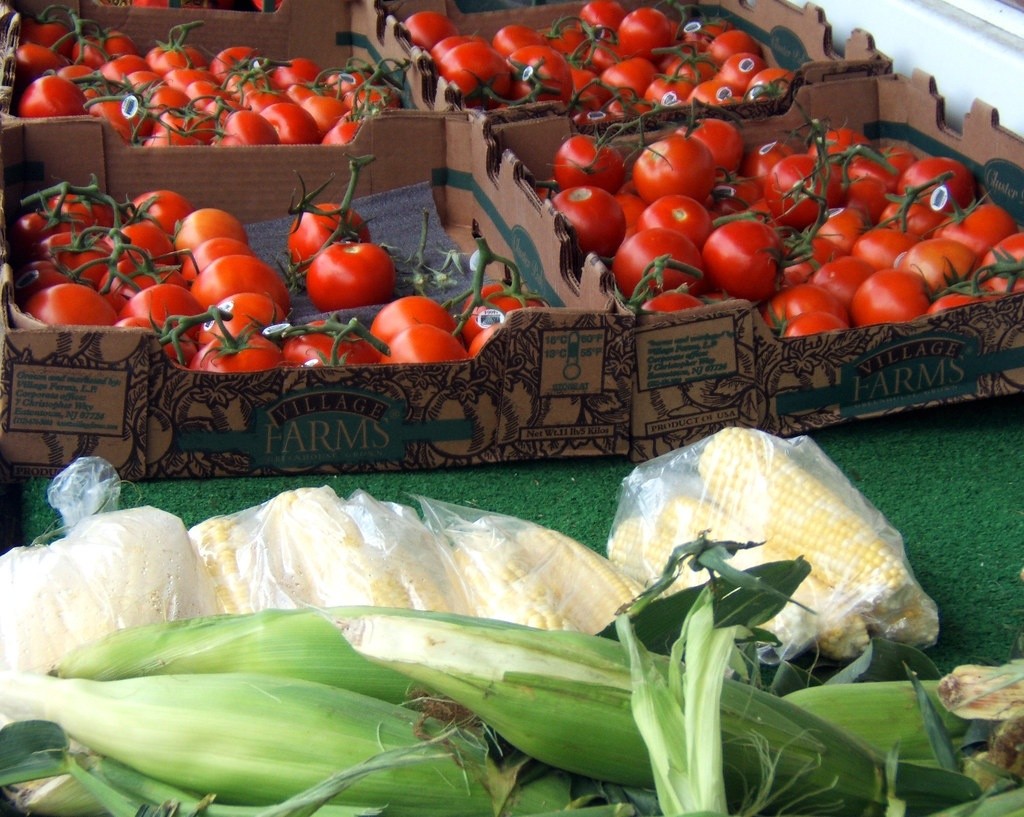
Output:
[0,1,1024,484]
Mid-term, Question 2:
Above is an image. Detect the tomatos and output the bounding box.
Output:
[10,0,1024,371]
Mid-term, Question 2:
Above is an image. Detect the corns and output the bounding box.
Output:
[0,424,1023,817]
